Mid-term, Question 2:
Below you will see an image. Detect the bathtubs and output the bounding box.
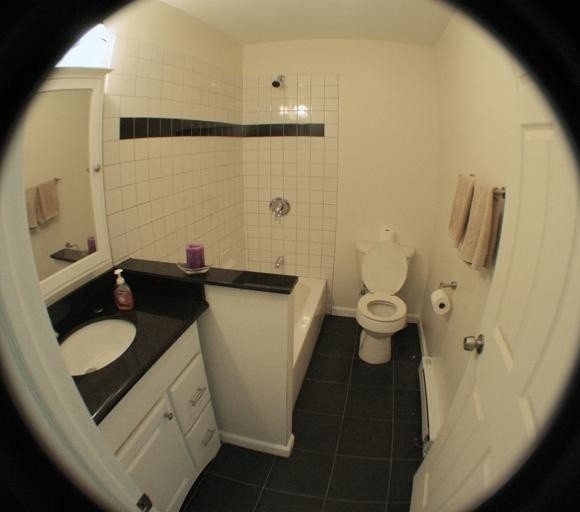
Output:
[293,282,312,326]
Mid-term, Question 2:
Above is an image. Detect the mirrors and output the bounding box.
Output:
[20,74,109,298]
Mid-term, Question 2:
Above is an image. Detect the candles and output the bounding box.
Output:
[186,243,205,268]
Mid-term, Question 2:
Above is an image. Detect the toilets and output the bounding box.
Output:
[356,241,407,365]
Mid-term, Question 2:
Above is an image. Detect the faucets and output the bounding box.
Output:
[55,331,61,339]
[275,256,284,268]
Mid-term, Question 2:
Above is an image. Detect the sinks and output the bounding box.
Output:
[59,317,137,377]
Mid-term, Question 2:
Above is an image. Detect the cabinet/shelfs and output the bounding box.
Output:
[115,351,223,511]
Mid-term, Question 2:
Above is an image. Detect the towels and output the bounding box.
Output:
[444,174,502,272]
[26,180,61,231]
